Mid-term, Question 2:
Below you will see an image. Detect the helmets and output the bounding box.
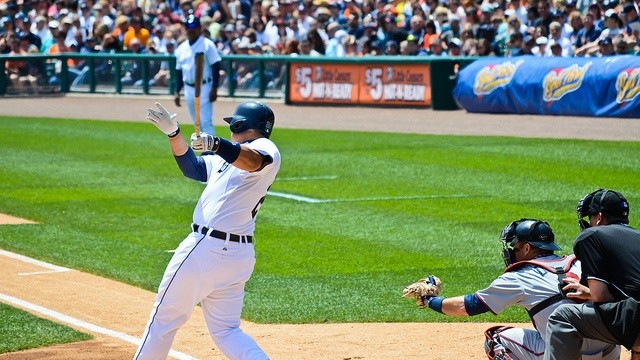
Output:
[222,103,274,134]
[577,188,628,230]
[498,217,561,267]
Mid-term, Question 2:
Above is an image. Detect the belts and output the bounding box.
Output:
[185,78,211,86]
[192,224,252,242]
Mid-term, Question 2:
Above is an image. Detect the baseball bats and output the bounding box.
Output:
[195,51,204,138]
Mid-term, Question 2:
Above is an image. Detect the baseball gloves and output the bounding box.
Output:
[402,273,441,309]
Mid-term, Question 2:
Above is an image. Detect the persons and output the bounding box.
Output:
[199,0,291,91]
[174,14,222,136]
[131,100,282,360]
[544,188,639,359]
[418,219,620,359]
[0,2,173,87]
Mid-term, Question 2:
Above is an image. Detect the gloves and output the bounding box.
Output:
[191,132,219,154]
[146,102,180,137]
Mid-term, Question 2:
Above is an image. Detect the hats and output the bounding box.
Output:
[61,17,72,24]
[116,15,127,25]
[314,7,330,20]
[223,24,233,30]
[48,19,60,27]
[624,3,634,12]
[605,9,615,16]
[59,7,68,14]
[183,17,199,24]
[35,16,47,21]
